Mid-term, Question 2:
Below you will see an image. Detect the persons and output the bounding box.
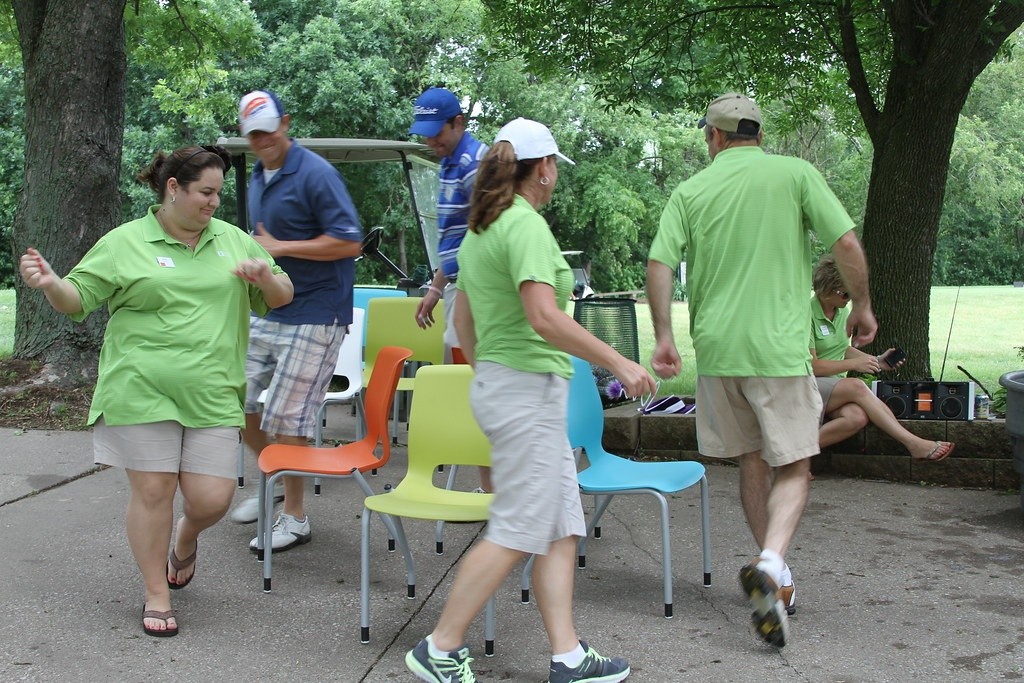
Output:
[408,88,491,493]
[646,92,878,647]
[19,146,294,638]
[405,117,658,683]
[232,91,363,553]
[808,258,956,461]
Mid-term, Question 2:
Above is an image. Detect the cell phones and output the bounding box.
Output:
[884,346,907,368]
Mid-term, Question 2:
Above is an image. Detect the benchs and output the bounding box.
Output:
[600,289,644,300]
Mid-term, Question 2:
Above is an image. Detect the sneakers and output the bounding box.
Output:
[740,557,789,649]
[405,635,478,683]
[780,580,796,614]
[547,640,630,683]
[249,509,312,552]
[232,478,284,524]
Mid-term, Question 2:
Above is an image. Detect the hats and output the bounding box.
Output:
[697,93,762,139]
[494,117,575,166]
[238,90,284,137]
[408,87,461,137]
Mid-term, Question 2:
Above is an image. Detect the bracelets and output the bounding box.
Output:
[429,286,442,295]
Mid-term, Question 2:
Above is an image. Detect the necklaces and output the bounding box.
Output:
[185,234,199,247]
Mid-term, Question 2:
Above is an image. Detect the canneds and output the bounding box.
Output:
[975,393,989,419]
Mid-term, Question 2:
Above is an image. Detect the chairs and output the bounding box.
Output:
[236,285,710,659]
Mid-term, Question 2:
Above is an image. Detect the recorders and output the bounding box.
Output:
[872,285,975,421]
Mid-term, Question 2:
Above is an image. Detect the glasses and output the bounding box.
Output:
[832,287,848,300]
[705,134,712,143]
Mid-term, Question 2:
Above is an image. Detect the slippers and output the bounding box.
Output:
[913,440,955,464]
[165,536,197,589]
[142,603,178,637]
